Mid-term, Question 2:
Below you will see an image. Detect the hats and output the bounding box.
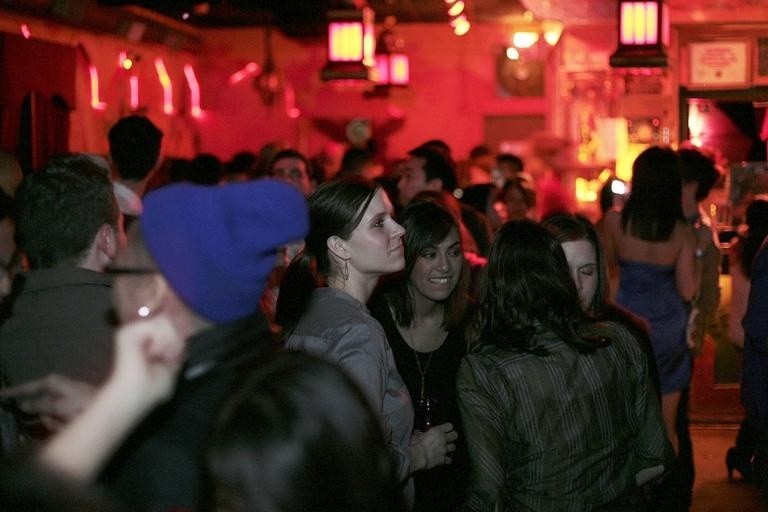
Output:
[140,180,313,327]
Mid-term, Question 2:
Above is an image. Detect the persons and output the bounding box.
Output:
[2,116,767,512]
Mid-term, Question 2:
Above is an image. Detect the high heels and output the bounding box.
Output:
[725,445,768,483]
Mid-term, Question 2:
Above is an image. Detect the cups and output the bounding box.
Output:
[418,398,440,431]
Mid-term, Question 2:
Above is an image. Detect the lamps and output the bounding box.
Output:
[608,1,669,67]
[365,50,411,98]
[320,7,371,80]
